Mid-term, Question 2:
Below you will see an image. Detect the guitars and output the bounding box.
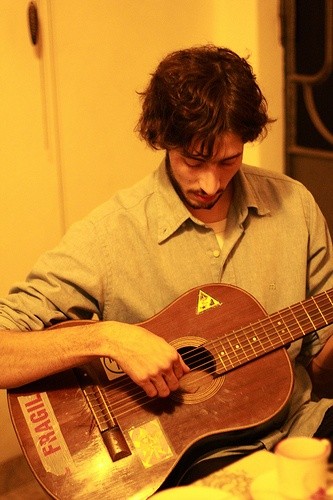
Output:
[6,283,333,500]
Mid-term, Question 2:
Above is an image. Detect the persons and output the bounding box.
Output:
[0,45,333,465]
